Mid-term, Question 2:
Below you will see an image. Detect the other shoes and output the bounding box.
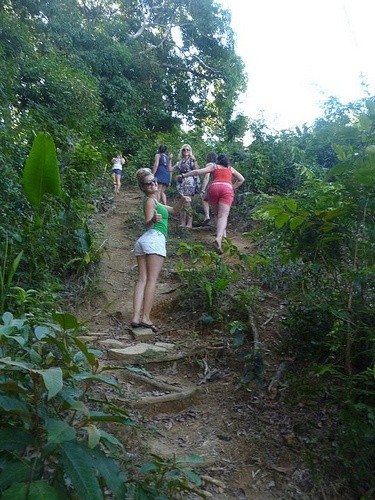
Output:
[212,242,223,253]
[201,219,210,225]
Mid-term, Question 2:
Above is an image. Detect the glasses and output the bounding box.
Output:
[182,149,190,151]
[143,178,157,187]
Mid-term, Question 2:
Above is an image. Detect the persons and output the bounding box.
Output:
[131,168,191,332]
[199,151,217,224]
[169,144,200,229]
[152,144,171,205]
[111,150,125,195]
[177,154,245,254]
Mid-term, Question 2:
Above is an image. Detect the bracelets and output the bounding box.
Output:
[151,218,156,224]
[182,173,185,179]
[201,189,205,194]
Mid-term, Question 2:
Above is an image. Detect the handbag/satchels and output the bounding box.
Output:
[196,175,201,193]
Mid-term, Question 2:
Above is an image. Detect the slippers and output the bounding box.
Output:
[140,322,158,332]
[131,322,140,328]
